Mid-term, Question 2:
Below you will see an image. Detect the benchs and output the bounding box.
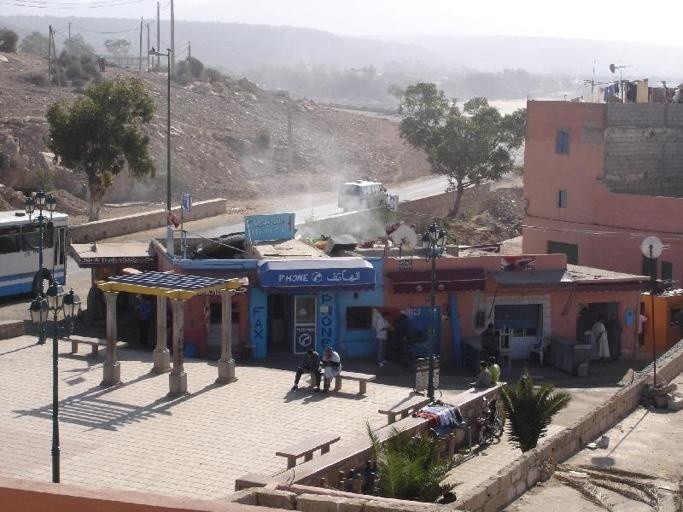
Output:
[275,429,342,468]
[317,368,378,394]
[61,334,129,360]
[378,394,431,426]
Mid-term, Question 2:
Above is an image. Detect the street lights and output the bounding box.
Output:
[24,190,57,290]
[148,46,176,258]
[422,219,449,404]
[28,278,82,484]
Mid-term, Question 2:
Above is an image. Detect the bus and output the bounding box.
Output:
[0,209,72,299]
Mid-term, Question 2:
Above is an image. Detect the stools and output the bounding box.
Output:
[492,323,514,377]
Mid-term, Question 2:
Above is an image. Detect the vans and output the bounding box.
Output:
[338,179,386,212]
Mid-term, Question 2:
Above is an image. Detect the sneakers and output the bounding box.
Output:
[376,359,389,366]
[291,386,338,394]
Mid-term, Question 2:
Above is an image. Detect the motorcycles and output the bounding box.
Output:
[474,394,503,448]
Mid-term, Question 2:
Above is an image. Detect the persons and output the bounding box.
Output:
[374,312,396,368]
[467,360,492,389]
[320,346,342,394]
[638,310,647,345]
[488,355,500,384]
[289,348,321,393]
[480,322,498,361]
[590,317,609,361]
[136,297,154,349]
[604,312,623,361]
